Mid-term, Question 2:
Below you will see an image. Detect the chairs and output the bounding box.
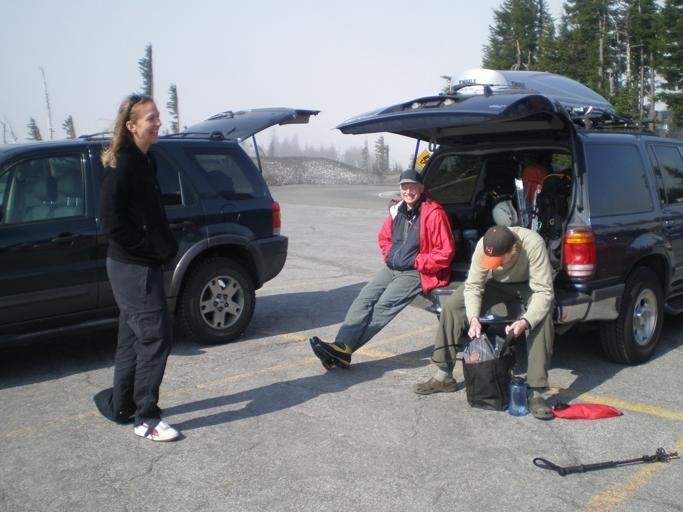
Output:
[49,170,84,220]
[17,177,55,222]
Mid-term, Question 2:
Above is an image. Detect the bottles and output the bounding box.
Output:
[508,376,527,415]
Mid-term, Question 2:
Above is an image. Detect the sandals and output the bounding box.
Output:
[528,396,554,420]
[413,377,458,394]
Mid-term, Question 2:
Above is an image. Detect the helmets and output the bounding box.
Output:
[491,200,517,226]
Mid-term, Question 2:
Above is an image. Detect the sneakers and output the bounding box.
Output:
[318,341,352,368]
[136,421,180,442]
[309,336,335,369]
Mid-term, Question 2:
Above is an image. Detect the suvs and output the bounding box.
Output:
[335,65,682,365]
[1,107,323,353]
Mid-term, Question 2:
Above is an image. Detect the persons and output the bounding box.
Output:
[412,225,558,419]
[92,92,181,442]
[309,169,456,371]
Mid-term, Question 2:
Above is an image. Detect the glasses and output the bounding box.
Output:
[125,97,138,121]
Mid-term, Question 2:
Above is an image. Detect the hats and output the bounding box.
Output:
[399,170,424,185]
[481,227,515,268]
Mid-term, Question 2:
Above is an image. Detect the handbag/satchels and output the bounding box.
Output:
[472,185,511,225]
[522,165,549,203]
[462,351,510,411]
[537,175,570,240]
